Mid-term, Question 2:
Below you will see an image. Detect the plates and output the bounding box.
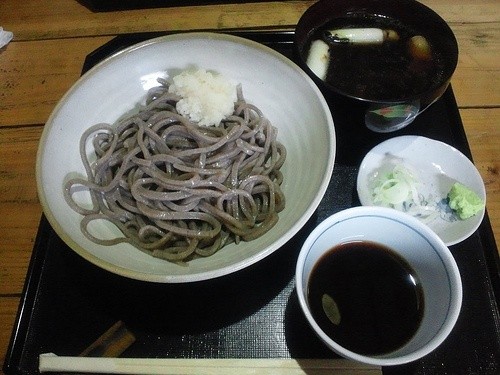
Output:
[356,133,487,249]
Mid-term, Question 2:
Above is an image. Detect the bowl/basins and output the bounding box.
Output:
[294,205,462,366]
[295,0,461,132]
[36,32,337,285]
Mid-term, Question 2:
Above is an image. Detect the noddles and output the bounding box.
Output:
[63,76,287,266]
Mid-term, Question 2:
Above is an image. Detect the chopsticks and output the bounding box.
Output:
[39,353,383,375]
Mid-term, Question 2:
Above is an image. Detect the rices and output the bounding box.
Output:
[167,70,236,127]
